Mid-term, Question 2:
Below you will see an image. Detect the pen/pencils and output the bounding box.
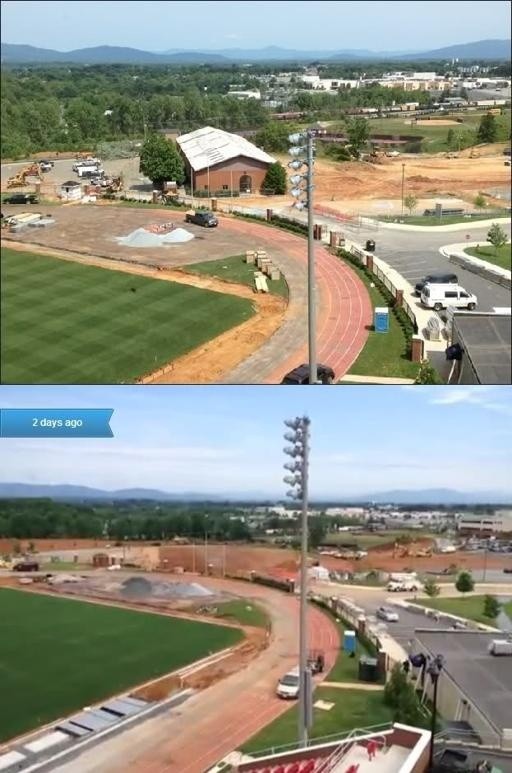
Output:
[275,663,300,701]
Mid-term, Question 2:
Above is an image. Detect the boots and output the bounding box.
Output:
[411,270,460,295]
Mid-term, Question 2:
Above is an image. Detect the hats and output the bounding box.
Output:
[12,559,40,571]
[376,604,399,622]
[504,155,511,166]
[502,566,512,573]
[280,360,337,385]
[1,191,41,204]
[107,564,122,570]
[29,154,115,186]
[496,86,501,90]
[431,531,512,558]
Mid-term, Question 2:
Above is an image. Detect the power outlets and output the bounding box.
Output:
[184,207,219,228]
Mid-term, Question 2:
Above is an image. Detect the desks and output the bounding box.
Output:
[280,411,314,747]
[405,649,447,772]
[123,534,129,568]
[444,341,466,384]
[283,125,322,383]
